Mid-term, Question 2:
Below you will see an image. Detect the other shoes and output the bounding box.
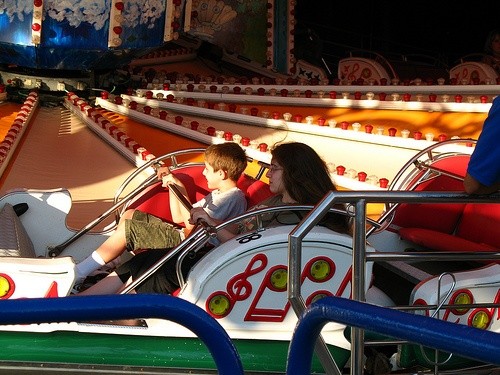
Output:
[71,283,82,294]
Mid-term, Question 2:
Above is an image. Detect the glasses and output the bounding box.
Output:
[268,166,284,174]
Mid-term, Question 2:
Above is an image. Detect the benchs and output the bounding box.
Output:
[384,152,500,264]
[120,159,280,297]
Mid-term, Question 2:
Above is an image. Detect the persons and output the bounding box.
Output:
[71,142,348,328]
[462,95,500,196]
[74,142,248,296]
